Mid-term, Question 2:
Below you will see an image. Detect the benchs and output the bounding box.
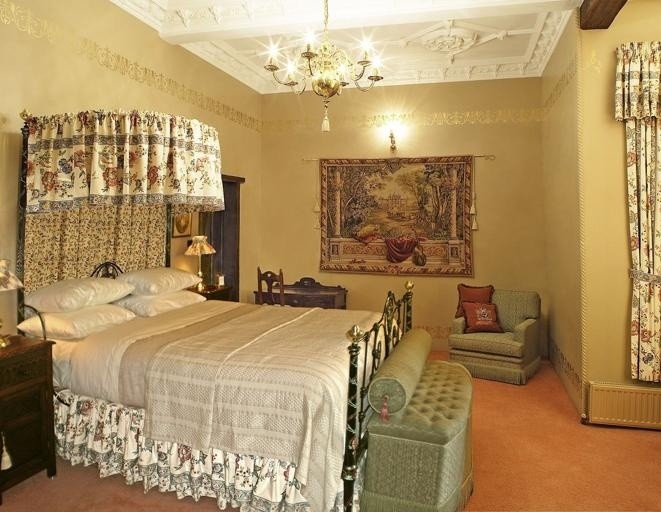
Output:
[360,359,481,511]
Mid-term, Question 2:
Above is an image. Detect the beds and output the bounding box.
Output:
[15,104,420,512]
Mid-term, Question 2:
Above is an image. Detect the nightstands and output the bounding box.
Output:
[0,330,62,508]
[191,284,234,301]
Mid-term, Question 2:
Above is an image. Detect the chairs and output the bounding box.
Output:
[256,265,287,305]
[447,284,546,388]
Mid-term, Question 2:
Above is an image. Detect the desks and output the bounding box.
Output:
[254,277,350,310]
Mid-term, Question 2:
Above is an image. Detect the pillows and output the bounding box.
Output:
[366,326,436,419]
[16,306,137,343]
[461,300,505,334]
[456,281,497,317]
[112,268,204,292]
[116,288,208,316]
[20,275,138,312]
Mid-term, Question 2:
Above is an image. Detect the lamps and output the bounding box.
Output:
[0,258,25,348]
[183,234,218,290]
[385,129,401,151]
[259,0,382,138]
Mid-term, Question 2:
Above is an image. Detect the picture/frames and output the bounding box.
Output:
[171,209,194,239]
[318,155,478,279]
